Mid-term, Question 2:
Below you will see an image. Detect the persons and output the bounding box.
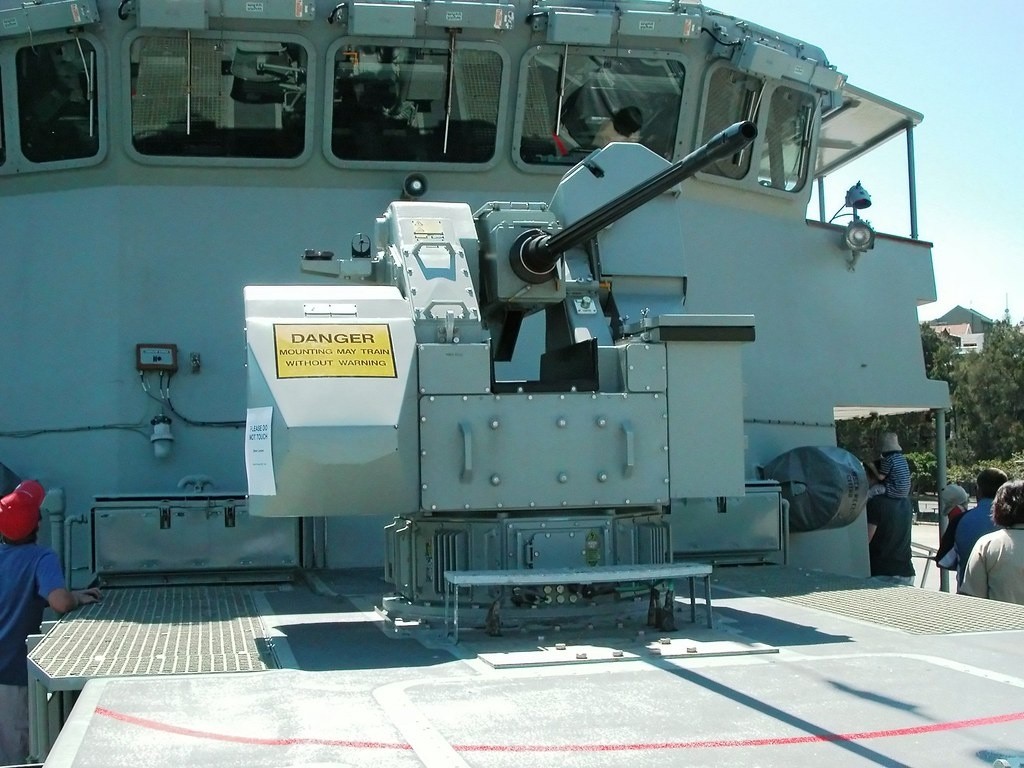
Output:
[0,482,103,768]
[864,434,1024,606]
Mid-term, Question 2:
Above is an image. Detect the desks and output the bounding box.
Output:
[442,561,715,646]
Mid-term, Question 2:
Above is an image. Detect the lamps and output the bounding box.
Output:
[149,414,175,462]
[845,219,876,254]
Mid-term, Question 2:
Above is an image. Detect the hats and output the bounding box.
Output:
[1,479,46,539]
[880,433,904,453]
[942,484,970,516]
